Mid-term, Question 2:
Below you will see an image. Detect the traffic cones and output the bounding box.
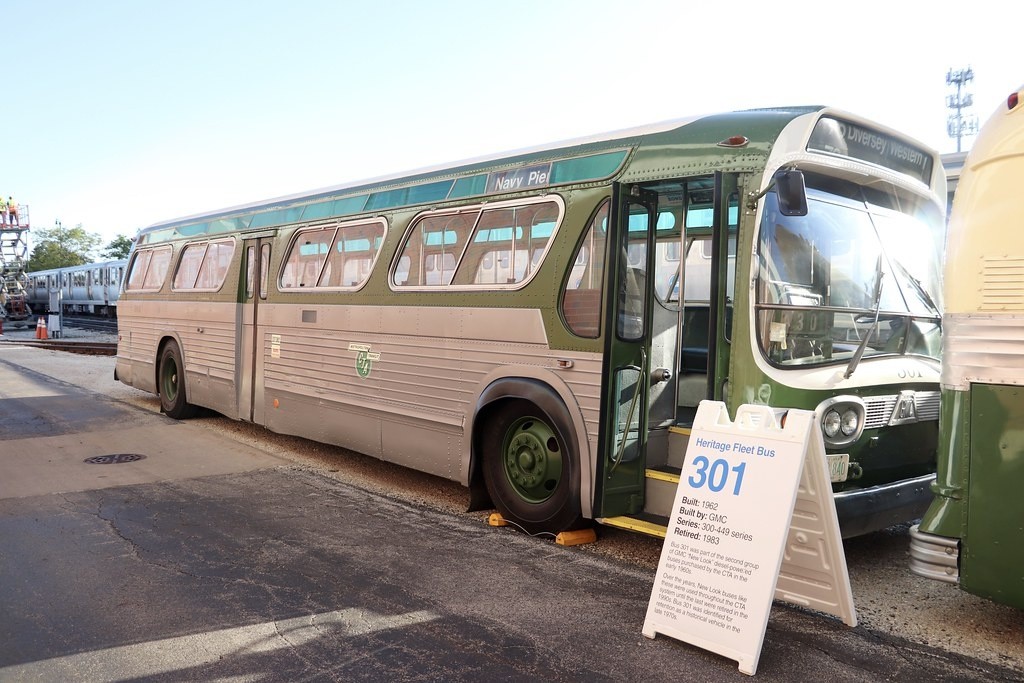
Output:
[40,316,49,340]
[31,316,42,340]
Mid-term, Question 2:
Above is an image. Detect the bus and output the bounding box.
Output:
[908,84,1024,615]
[112,104,948,544]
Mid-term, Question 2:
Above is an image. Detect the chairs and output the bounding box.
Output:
[785,285,875,363]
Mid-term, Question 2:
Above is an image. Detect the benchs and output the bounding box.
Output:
[562,289,601,337]
[681,305,732,375]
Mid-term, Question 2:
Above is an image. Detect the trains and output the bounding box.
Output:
[22,202,740,372]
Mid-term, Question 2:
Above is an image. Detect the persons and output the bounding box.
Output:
[0,197,8,228]
[7,196,19,226]
[0,288,6,337]
